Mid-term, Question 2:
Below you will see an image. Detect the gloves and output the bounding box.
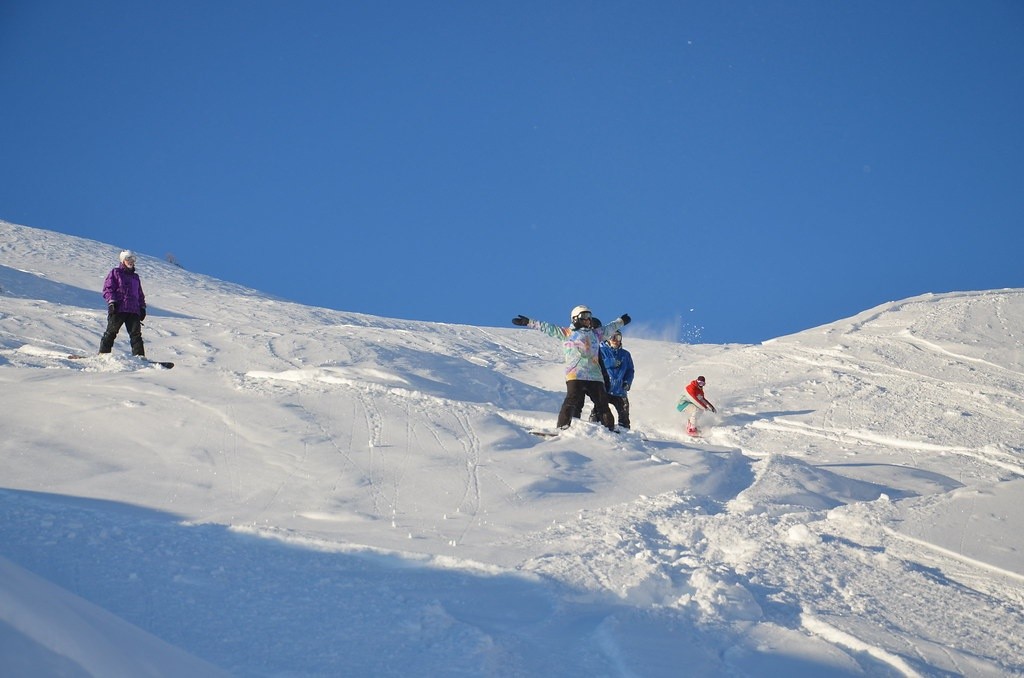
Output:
[621,314,631,325]
[623,383,630,392]
[616,360,621,367]
[512,315,529,326]
[108,302,115,314]
[140,308,146,321]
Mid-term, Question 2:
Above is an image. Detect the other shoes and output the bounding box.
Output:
[688,428,699,437]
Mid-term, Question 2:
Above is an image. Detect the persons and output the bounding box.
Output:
[96,249,146,361]
[510,303,635,436]
[675,376,717,438]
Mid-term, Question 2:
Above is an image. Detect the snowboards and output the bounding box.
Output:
[685,428,701,440]
[68,355,174,370]
[529,431,562,437]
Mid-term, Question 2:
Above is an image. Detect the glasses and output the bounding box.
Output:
[697,380,705,387]
[125,256,136,263]
[576,312,592,321]
[609,333,622,342]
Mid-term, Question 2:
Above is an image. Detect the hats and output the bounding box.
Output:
[120,250,134,262]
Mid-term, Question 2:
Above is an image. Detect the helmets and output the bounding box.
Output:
[571,305,591,323]
[588,318,602,329]
